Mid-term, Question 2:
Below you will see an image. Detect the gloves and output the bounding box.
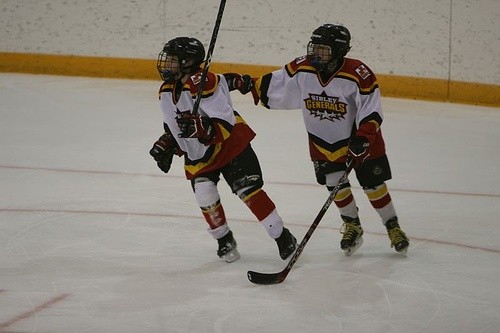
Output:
[223,73,246,92]
[346,136,371,170]
[149,135,173,174]
[176,114,214,139]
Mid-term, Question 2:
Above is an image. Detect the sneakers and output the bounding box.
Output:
[385,216,410,257]
[339,213,364,257]
[216,229,241,264]
[274,227,300,260]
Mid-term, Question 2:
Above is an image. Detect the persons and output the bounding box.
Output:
[222,23,410,253]
[149,37,297,260]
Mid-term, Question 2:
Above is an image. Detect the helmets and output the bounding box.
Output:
[306,22,352,68]
[155,35,206,84]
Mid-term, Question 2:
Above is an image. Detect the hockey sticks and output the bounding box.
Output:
[192,0,227,115]
[246,154,356,287]
